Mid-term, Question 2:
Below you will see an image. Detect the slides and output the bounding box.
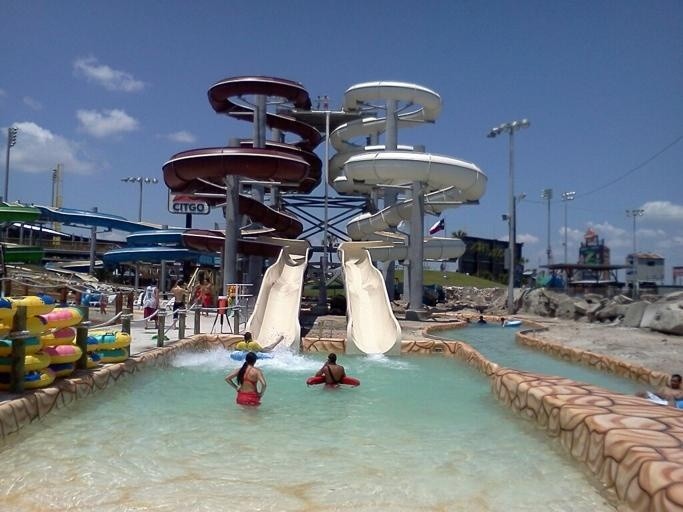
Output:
[330,81,489,358]
[163,77,323,355]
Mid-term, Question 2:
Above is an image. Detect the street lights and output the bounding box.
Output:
[486,118,529,314]
[625,209,644,298]
[560,191,575,260]
[121,177,158,222]
[4,127,18,202]
[539,188,553,265]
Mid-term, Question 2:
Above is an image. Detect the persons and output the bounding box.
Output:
[477,315,487,323]
[98,288,107,315]
[236,332,284,353]
[315,353,346,386]
[635,374,683,408]
[225,351,266,406]
[140,280,160,329]
[194,277,213,316]
[314,95,329,111]
[171,278,191,330]
[501,317,509,328]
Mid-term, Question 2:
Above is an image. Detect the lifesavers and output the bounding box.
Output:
[308,377,359,388]
[502,320,522,326]
[646,392,683,409]
[0,296,131,390]
[230,351,273,361]
